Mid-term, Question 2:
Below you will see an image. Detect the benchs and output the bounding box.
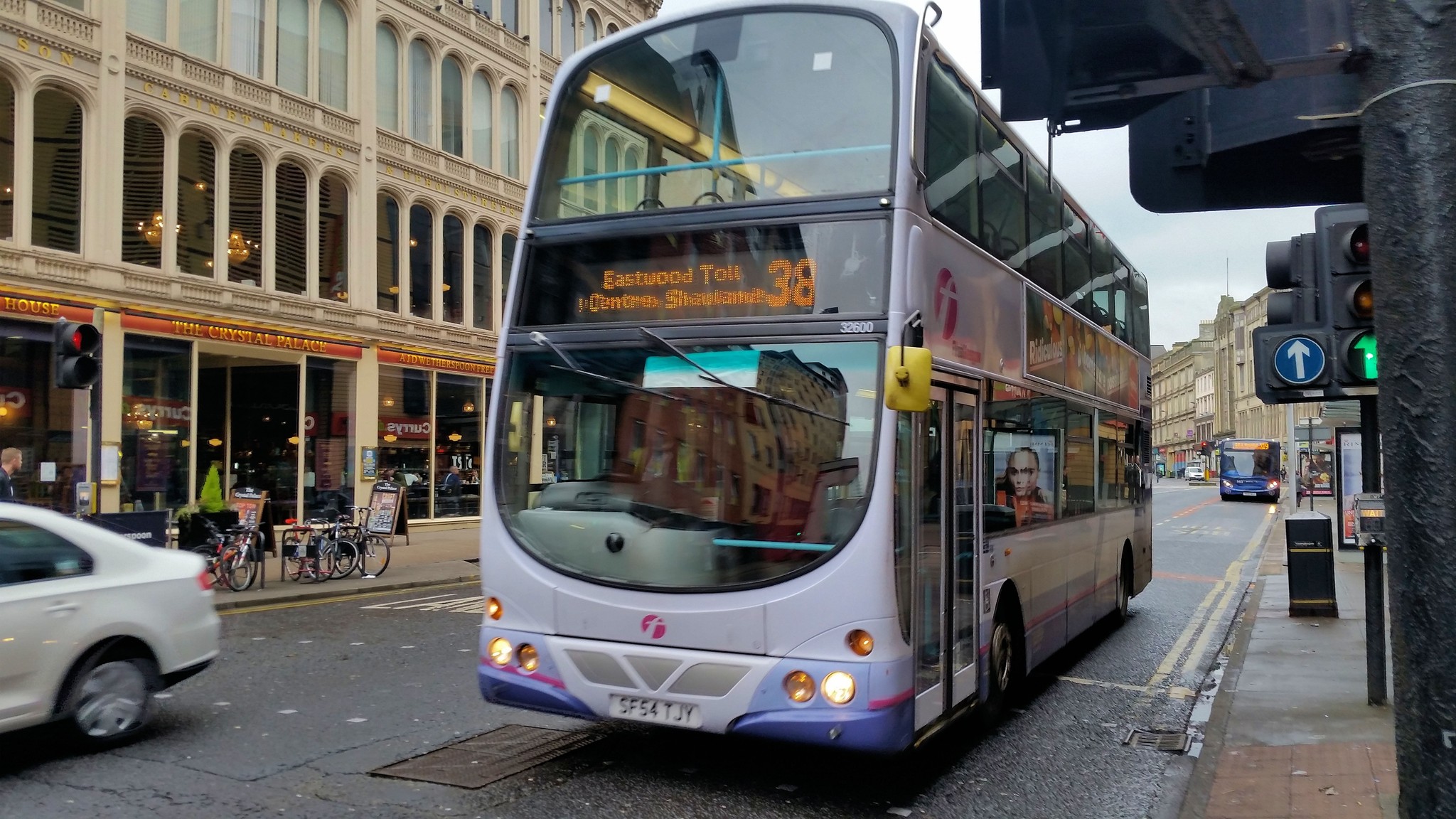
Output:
[407,494,480,517]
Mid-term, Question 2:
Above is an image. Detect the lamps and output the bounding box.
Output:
[1160,410,1165,412]
[383,433,397,443]
[383,396,394,406]
[448,431,462,442]
[227,230,251,265]
[193,180,207,191]
[463,401,474,412]
[1190,401,1195,404]
[137,211,181,245]
[546,415,556,426]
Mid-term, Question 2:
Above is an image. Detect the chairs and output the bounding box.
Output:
[1073,288,1085,316]
[1093,301,1102,327]
[635,197,665,212]
[946,200,970,232]
[982,219,999,252]
[997,235,1020,260]
[1092,307,1109,327]
[1116,318,1124,341]
[693,191,724,206]
[1118,320,1125,342]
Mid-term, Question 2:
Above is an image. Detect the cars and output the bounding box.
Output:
[1185,466,1205,481]
[0,503,217,758]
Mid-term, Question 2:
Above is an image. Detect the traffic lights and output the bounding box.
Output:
[1314,203,1388,389]
[1201,440,1208,456]
[1262,231,1322,327]
[54,317,102,395]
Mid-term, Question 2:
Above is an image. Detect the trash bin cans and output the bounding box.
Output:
[1284,510,1338,616]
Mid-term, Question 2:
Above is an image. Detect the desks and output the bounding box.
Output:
[460,482,480,516]
[406,484,451,518]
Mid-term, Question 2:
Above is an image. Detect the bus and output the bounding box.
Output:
[471,0,1153,767]
[1215,438,1288,503]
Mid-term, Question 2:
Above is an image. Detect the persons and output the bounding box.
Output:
[384,468,408,496]
[0,447,26,502]
[1156,468,1160,484]
[1180,466,1185,478]
[1281,466,1288,482]
[1304,454,1331,484]
[439,466,462,517]
[994,447,1056,528]
[465,468,479,514]
[1295,469,1304,508]
[1060,465,1070,490]
[1122,455,1145,505]
[1152,466,1155,474]
[612,409,711,498]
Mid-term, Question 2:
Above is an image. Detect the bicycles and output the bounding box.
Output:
[282,503,392,583]
[190,521,271,591]
